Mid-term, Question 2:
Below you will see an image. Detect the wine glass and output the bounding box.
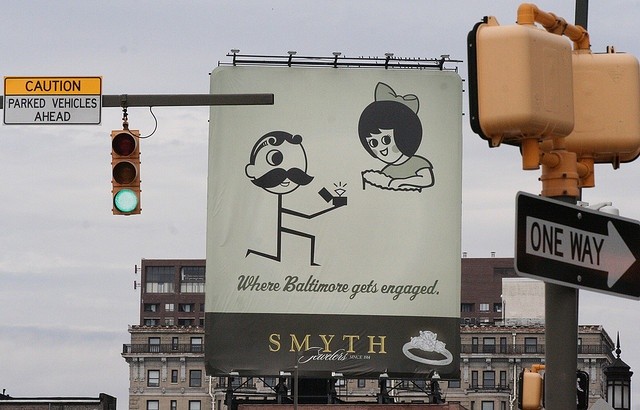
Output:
[403,330,453,366]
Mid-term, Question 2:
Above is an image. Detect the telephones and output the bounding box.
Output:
[4,77,102,124]
[514,192,639,301]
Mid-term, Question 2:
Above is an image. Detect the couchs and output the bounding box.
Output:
[111,129,140,214]
